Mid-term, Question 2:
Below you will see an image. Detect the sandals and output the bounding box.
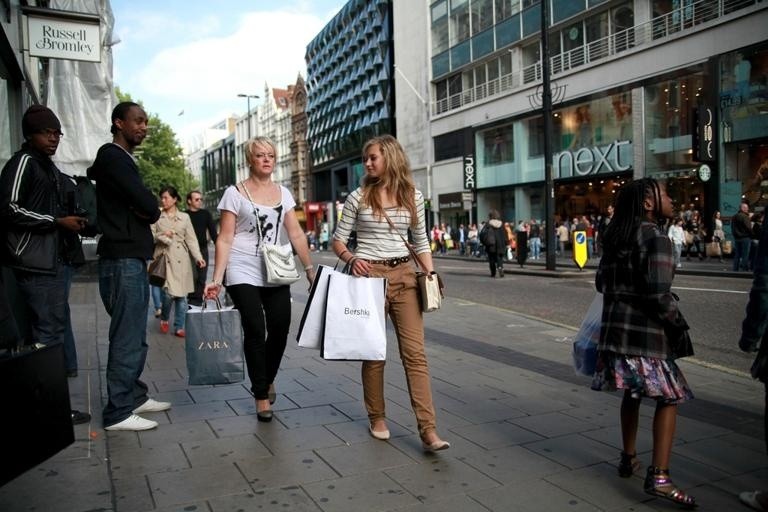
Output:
[616,450,641,477]
[644,466,700,507]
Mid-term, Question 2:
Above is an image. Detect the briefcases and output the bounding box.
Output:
[0,341,75,486]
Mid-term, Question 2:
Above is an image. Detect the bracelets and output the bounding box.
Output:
[212,280,223,287]
[304,265,313,271]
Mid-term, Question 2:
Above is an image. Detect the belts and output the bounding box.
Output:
[361,255,410,268]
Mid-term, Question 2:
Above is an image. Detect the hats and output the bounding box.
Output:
[22,104,61,137]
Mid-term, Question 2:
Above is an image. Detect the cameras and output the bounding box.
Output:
[60,191,96,237]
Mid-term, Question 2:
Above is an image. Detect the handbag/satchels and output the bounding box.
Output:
[184,294,245,386]
[295,250,354,350]
[320,257,387,362]
[416,271,445,312]
[722,240,732,253]
[706,239,721,256]
[148,251,167,288]
[683,228,694,243]
[262,242,301,285]
[480,224,495,247]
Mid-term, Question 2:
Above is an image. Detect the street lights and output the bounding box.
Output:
[236,92,260,139]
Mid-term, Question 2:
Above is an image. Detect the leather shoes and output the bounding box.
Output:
[256,401,273,421]
[154,307,162,318]
[269,384,276,404]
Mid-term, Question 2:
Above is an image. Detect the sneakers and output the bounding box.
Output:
[423,441,450,451]
[160,320,169,334]
[498,267,504,277]
[72,410,91,424]
[739,489,762,510]
[370,424,390,441]
[103,415,158,432]
[175,328,185,338]
[132,398,171,412]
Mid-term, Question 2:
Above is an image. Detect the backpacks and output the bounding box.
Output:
[68,167,104,238]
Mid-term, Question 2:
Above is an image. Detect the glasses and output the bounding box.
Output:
[196,198,203,201]
[41,128,63,138]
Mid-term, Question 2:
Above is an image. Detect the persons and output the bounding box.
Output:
[739,205,768,508]
[85,102,171,431]
[1,104,88,424]
[330,133,450,452]
[663,203,762,272]
[202,136,318,421]
[305,228,328,252]
[596,178,698,507]
[147,186,220,338]
[429,203,615,277]
